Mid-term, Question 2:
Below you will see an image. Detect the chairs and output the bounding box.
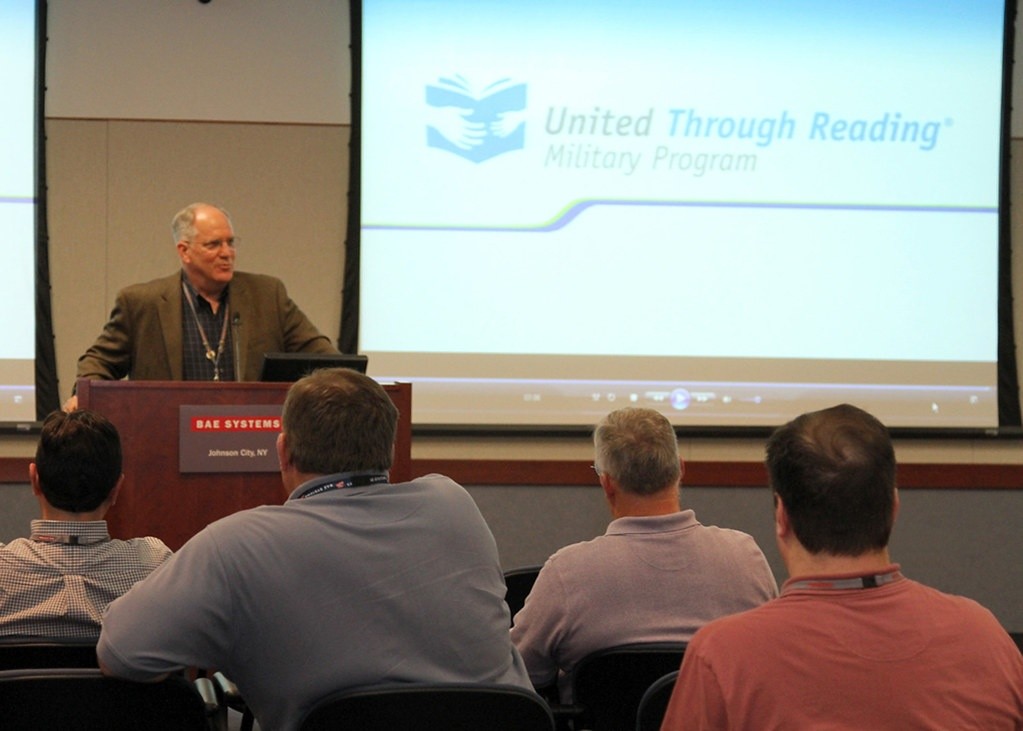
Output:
[294,681,556,731]
[503,566,543,629]
[0,633,254,731]
[536,641,688,731]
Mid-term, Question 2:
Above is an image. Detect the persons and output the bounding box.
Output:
[59,202,345,414]
[658,404,1023,731]
[0,409,174,639]
[508,407,803,709]
[96,368,537,731]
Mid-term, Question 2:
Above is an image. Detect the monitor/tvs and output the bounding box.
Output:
[257,351,368,382]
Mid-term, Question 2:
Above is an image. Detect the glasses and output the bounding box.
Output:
[183,236,241,254]
